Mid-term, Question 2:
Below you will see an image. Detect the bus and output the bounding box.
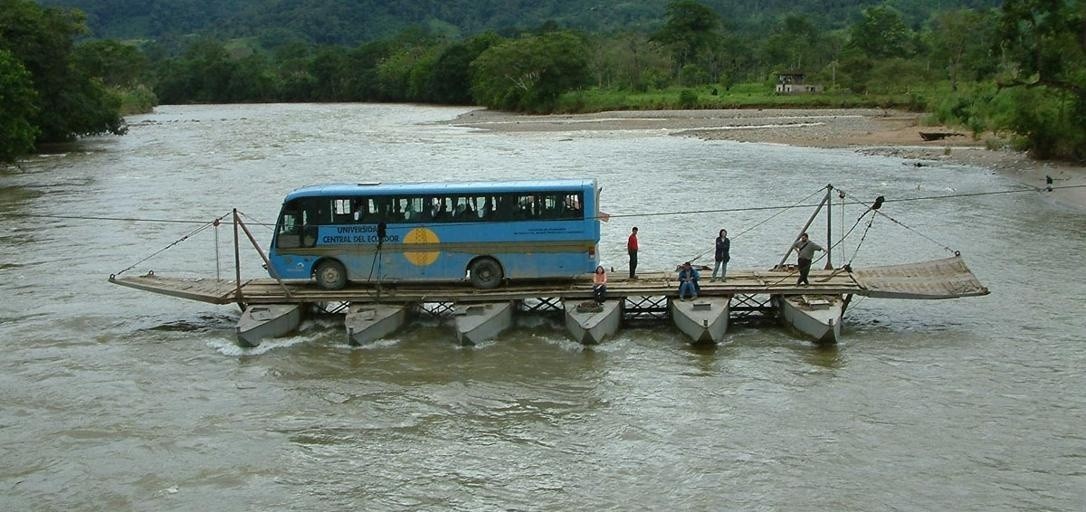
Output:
[262,179,603,289]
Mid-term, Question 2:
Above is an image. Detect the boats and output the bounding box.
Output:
[106,185,992,347]
[918,131,966,140]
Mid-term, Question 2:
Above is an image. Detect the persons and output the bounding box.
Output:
[627,227,639,277]
[592,266,607,303]
[791,233,826,288]
[677,262,701,302]
[709,229,731,282]
[1044,174,1053,192]
